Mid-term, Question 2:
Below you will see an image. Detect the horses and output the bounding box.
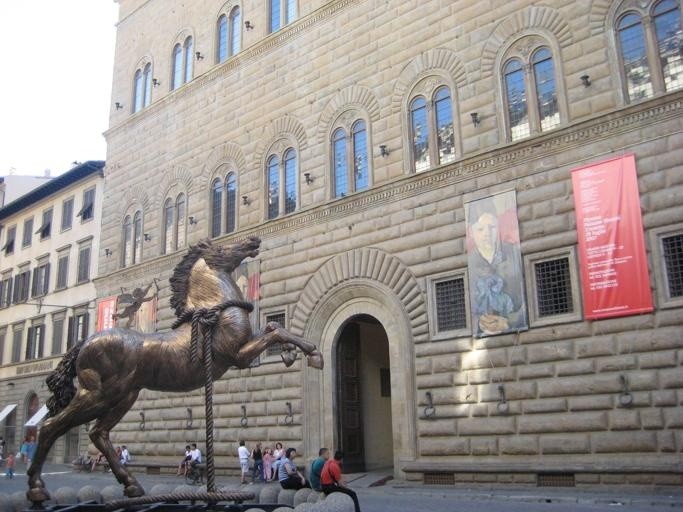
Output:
[26,235,323,503]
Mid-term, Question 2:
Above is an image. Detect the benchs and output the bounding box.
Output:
[81,460,306,476]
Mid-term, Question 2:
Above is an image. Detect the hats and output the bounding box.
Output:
[465,198,501,229]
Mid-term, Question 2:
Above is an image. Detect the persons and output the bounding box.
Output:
[0,435,5,466]
[4,454,15,480]
[175,445,192,477]
[308,448,330,492]
[110,282,158,329]
[270,442,285,481]
[237,440,251,484]
[78,444,131,475]
[19,436,27,465]
[465,197,527,335]
[249,442,263,485]
[183,443,202,478]
[319,450,362,512]
[277,448,311,490]
[24,435,38,480]
[261,447,276,483]
